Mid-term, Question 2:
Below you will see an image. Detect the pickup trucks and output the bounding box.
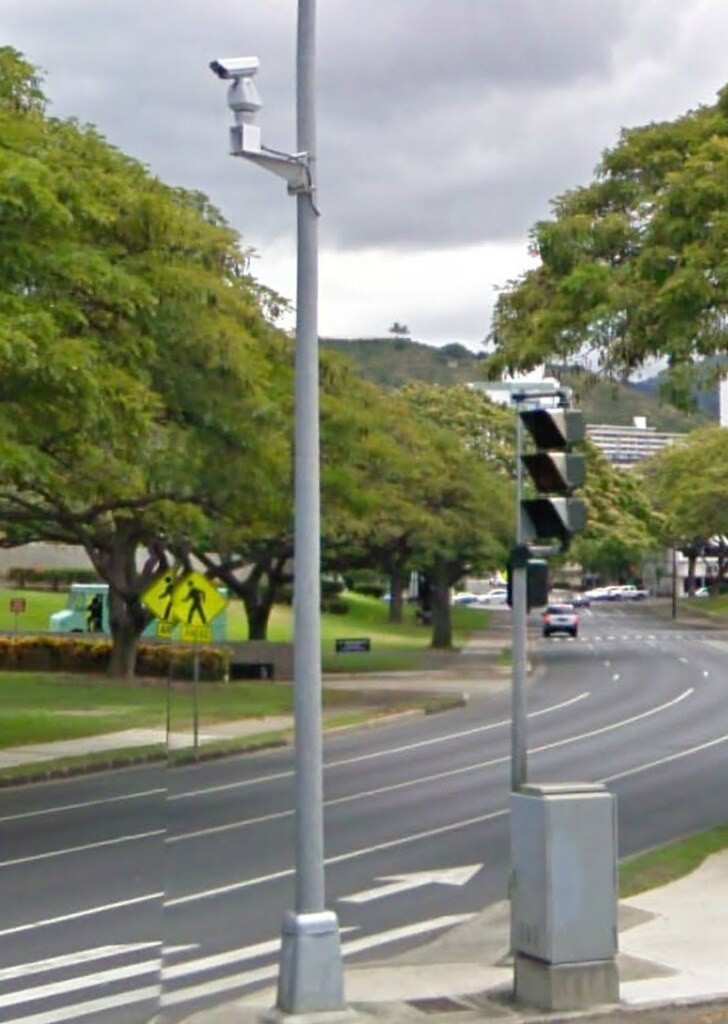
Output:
[609,584,650,602]
[543,605,579,638]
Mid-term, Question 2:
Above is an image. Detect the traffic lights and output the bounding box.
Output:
[519,404,594,544]
[501,553,551,608]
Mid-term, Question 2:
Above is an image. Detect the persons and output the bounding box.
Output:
[87,598,102,631]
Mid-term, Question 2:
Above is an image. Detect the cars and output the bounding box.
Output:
[695,587,711,598]
[454,593,478,605]
[479,589,509,606]
[552,585,608,605]
